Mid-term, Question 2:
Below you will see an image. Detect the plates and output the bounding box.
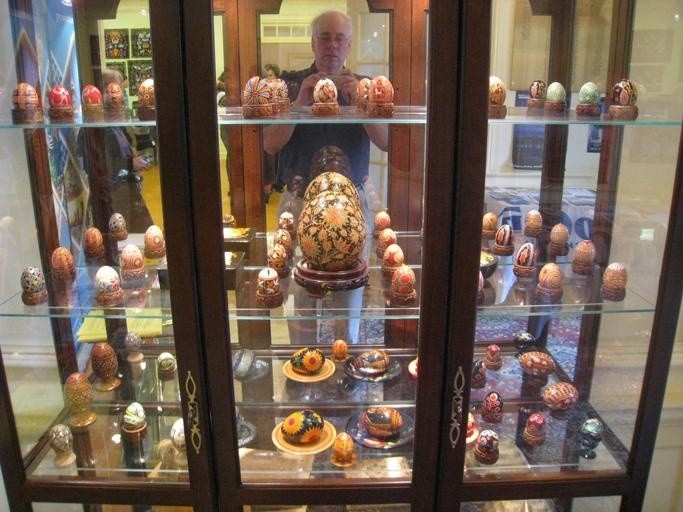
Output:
[271,354,415,468]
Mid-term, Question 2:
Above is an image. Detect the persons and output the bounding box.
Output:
[264,11,388,345]
[76,70,154,233]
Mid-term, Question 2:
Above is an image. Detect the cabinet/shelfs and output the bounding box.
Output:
[0,0,683,512]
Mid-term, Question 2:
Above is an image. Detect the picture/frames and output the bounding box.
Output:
[103,27,152,97]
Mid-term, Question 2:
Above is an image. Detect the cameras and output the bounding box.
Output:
[313,75,343,91]
[140,155,154,164]
